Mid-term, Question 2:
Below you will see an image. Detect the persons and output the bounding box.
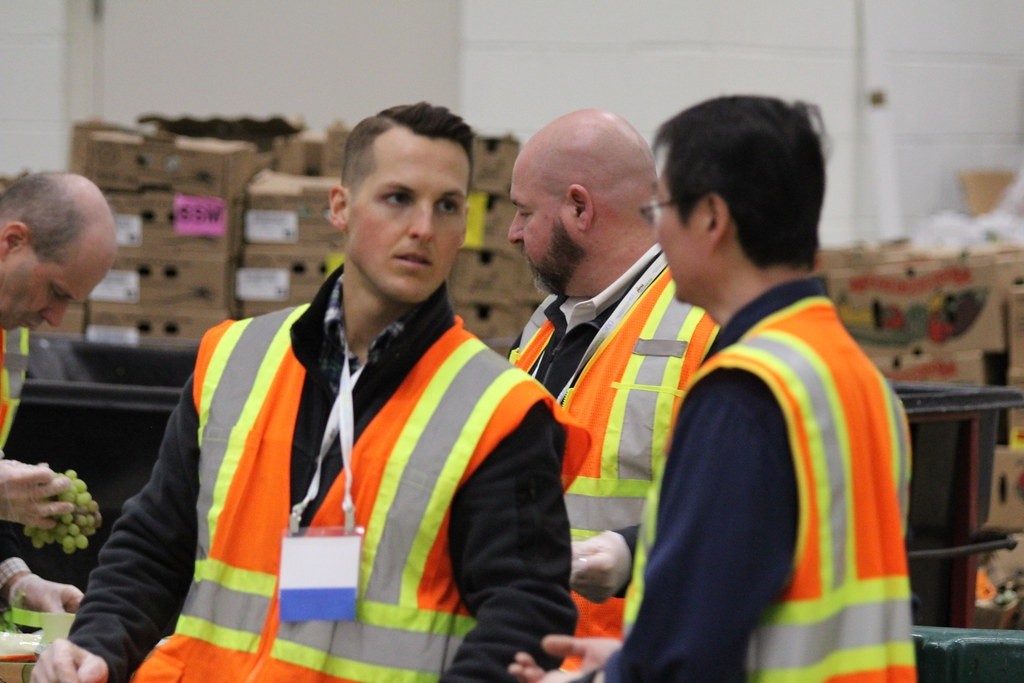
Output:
[27,104,578,683]
[0,170,116,632]
[505,94,917,683]
[504,104,721,672]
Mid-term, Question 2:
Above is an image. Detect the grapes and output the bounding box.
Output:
[23,469,101,553]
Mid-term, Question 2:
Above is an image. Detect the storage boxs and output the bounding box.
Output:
[33,115,1024,630]
[0,661,37,683]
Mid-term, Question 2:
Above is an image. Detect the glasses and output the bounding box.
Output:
[640,200,676,227]
